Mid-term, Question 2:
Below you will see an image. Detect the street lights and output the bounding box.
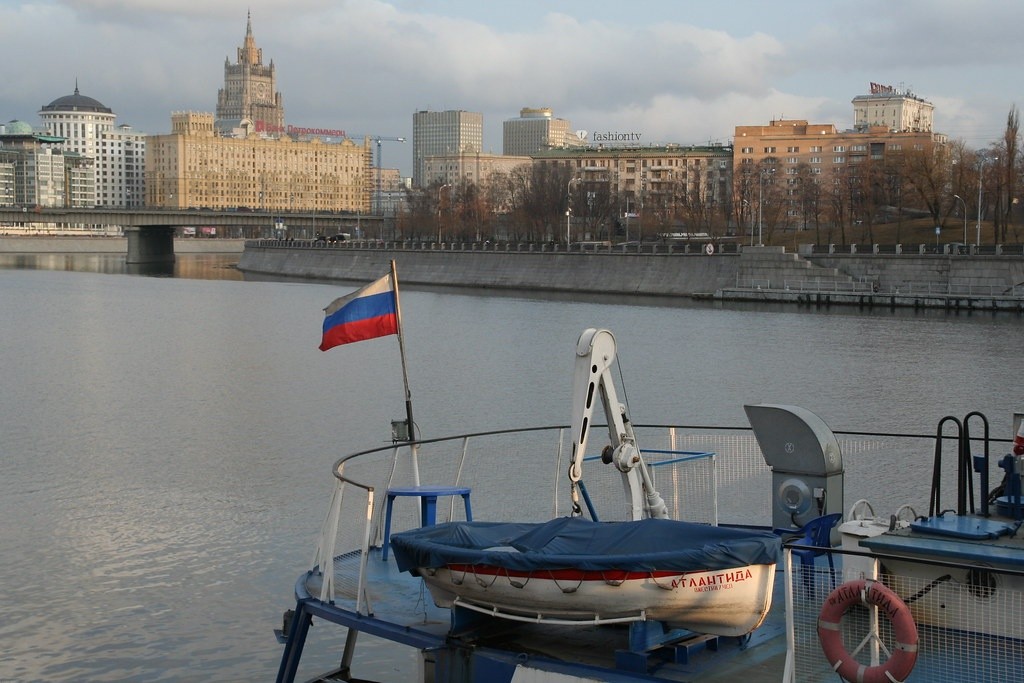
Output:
[954,194,966,245]
[759,168,776,244]
[567,178,581,252]
[977,156,999,254]
[438,184,452,244]
[743,199,754,247]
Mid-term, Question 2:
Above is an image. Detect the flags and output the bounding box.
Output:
[318,271,398,352]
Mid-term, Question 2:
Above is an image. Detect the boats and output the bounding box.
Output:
[275,328,1024,682]
[390,517,783,637]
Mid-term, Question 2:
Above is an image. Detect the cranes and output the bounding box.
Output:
[309,134,407,215]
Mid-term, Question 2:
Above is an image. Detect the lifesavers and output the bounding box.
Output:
[820,579,918,683]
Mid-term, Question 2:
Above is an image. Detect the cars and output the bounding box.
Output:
[327,234,351,245]
[319,210,333,215]
[340,210,352,215]
[933,242,967,255]
[237,207,254,213]
[199,207,213,212]
